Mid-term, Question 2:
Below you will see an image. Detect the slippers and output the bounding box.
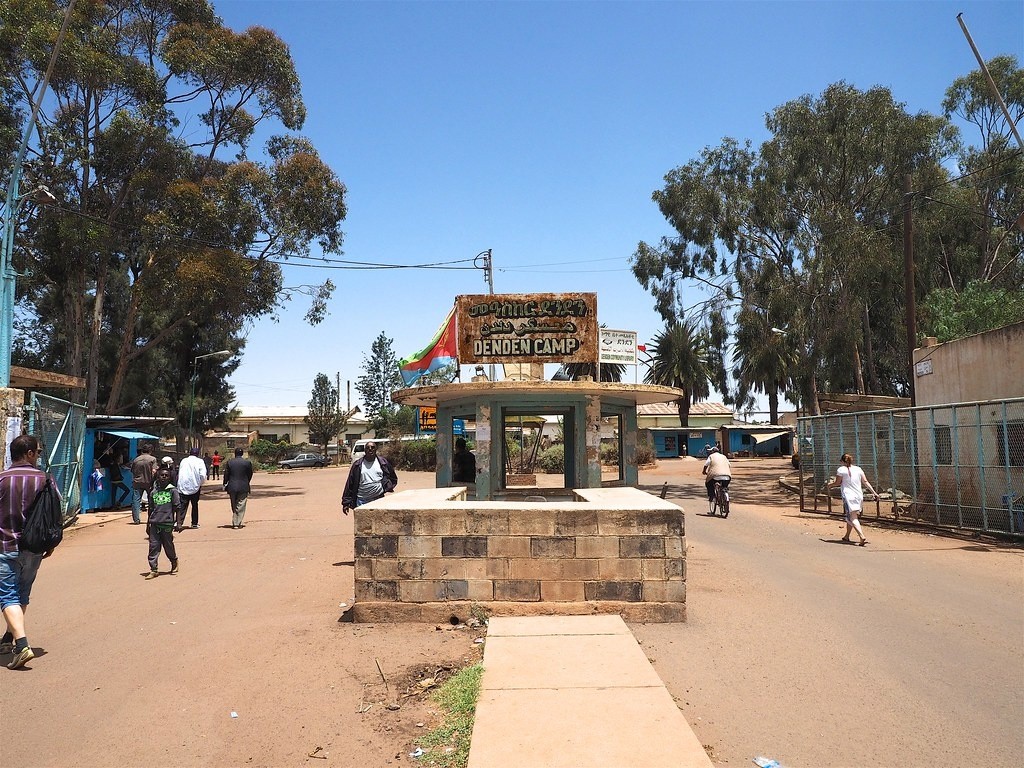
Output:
[842,537,850,544]
[859,541,868,546]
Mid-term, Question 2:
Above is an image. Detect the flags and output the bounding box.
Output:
[398,306,458,388]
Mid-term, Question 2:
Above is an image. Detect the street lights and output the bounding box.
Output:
[188,350,230,455]
[772,327,799,418]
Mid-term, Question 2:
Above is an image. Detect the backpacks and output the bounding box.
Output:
[18,473,63,554]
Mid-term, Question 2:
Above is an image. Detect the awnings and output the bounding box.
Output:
[96,430,162,462]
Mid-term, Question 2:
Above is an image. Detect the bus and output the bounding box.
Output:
[350,435,431,465]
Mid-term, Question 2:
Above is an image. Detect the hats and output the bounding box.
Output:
[707,447,719,452]
[161,455,174,466]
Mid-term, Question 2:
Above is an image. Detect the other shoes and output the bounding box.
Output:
[708,495,715,502]
[169,566,180,575]
[233,525,239,529]
[0,638,13,655]
[238,524,245,529]
[144,571,159,580]
[7,645,35,670]
[190,524,200,529]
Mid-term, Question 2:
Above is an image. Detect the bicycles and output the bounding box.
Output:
[703,473,730,517]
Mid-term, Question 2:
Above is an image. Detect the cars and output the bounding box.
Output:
[277,452,333,470]
[792,445,813,471]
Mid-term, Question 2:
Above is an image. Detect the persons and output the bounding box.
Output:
[341,441,397,516]
[702,442,711,459]
[223,449,253,529]
[175,448,207,530]
[453,439,475,483]
[0,435,62,670]
[715,441,721,452]
[131,444,157,524]
[682,442,687,458]
[144,468,181,579]
[702,447,732,513]
[212,450,220,480]
[101,448,130,509]
[828,454,879,546]
[160,457,178,486]
[203,453,212,480]
